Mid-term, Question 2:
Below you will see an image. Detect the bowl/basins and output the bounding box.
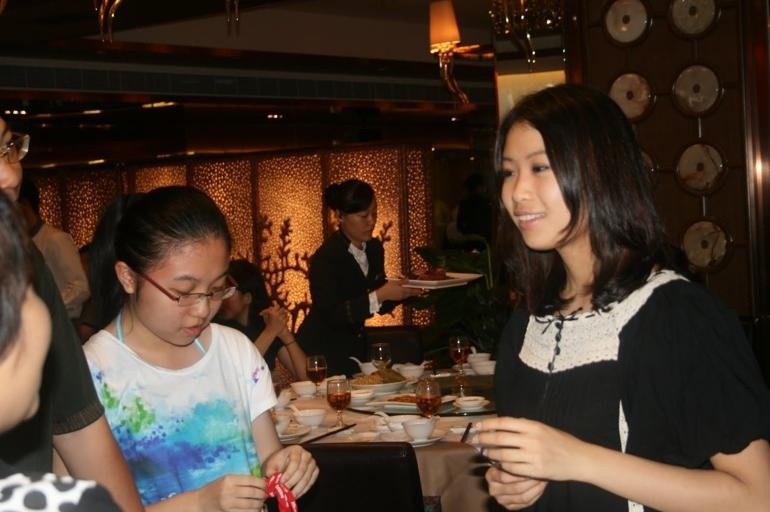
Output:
[452,353,496,376]
[351,363,457,413]
[369,415,441,448]
[270,381,325,442]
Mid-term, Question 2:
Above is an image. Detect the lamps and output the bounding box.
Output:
[425,0,481,104]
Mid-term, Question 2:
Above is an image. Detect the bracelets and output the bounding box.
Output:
[284,340,296,347]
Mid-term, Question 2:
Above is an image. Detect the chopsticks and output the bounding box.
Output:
[292,424,357,444]
[461,423,472,443]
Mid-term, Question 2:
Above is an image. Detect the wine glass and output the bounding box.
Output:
[372,343,391,370]
[415,381,441,417]
[450,337,469,382]
[328,379,351,428]
[306,356,327,399]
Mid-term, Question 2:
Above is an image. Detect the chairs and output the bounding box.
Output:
[262,440,426,511]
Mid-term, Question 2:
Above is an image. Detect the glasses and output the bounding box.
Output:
[0,132,30,163]
[135,267,240,306]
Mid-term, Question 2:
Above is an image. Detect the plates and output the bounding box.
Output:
[385,272,483,289]
[449,426,477,434]
[453,397,490,410]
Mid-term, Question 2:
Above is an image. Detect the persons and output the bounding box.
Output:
[296,178,427,380]
[75,184,320,512]
[210,258,309,381]
[0,187,122,512]
[76,192,148,344]
[0,118,143,512]
[18,180,92,327]
[469,82,770,512]
